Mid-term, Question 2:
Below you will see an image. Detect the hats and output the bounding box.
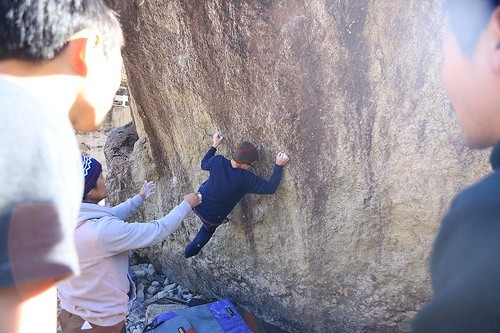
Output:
[233,142,258,164]
[82,156,102,199]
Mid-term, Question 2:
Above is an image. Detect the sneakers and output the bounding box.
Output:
[221,216,230,223]
[195,248,204,256]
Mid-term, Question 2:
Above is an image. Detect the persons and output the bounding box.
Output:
[184,132,290,258]
[409,0,500,333]
[57,152,203,333]
[0,0,126,333]
